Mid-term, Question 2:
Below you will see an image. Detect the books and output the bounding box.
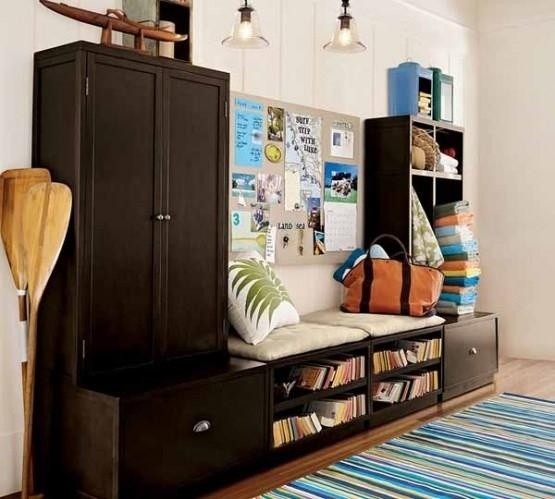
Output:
[418,101,428,108]
[418,108,431,114]
[269,336,444,451]
[419,96,431,104]
[419,91,432,98]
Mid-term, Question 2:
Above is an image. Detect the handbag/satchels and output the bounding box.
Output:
[341,233,444,317]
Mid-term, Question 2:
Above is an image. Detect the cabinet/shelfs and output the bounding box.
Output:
[32,356,268,497]
[228,337,371,487]
[32,39,229,386]
[371,323,444,430]
[436,312,499,403]
[362,115,466,265]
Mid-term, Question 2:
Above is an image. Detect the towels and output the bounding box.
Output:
[434,152,481,318]
[409,185,444,269]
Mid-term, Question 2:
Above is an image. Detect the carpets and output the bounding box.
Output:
[251,391,555,499]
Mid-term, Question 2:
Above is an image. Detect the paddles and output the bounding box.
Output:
[20,183,74,495]
[0,169,52,494]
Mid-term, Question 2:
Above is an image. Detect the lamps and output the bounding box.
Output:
[322,0,367,53]
[221,0,272,50]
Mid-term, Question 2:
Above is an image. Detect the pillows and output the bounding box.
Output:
[230,321,371,364]
[228,249,300,343]
[298,305,446,337]
